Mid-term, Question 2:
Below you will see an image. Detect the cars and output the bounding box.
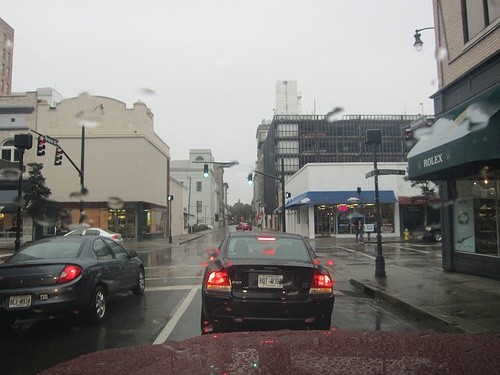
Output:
[62,227,124,246]
[200,231,336,335]
[235,223,252,232]
[0,234,147,325]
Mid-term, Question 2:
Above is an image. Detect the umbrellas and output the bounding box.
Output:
[346,213,365,218]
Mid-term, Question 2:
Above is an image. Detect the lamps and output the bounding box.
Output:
[414,26,434,50]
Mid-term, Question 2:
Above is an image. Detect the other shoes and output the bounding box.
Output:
[360,242,364,245]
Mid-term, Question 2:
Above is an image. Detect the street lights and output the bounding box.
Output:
[247,158,287,232]
[166,194,173,243]
[365,129,388,278]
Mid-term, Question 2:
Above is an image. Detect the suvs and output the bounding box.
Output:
[424,221,442,242]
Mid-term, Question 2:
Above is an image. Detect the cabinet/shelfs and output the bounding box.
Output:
[476,198,497,254]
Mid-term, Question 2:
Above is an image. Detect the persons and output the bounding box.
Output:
[356,218,364,245]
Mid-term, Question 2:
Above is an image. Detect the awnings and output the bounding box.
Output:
[273,205,283,215]
[285,190,397,209]
[406,84,500,179]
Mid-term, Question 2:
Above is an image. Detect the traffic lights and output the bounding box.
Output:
[36,136,46,157]
[204,164,209,179]
[54,147,63,166]
[247,173,252,186]
